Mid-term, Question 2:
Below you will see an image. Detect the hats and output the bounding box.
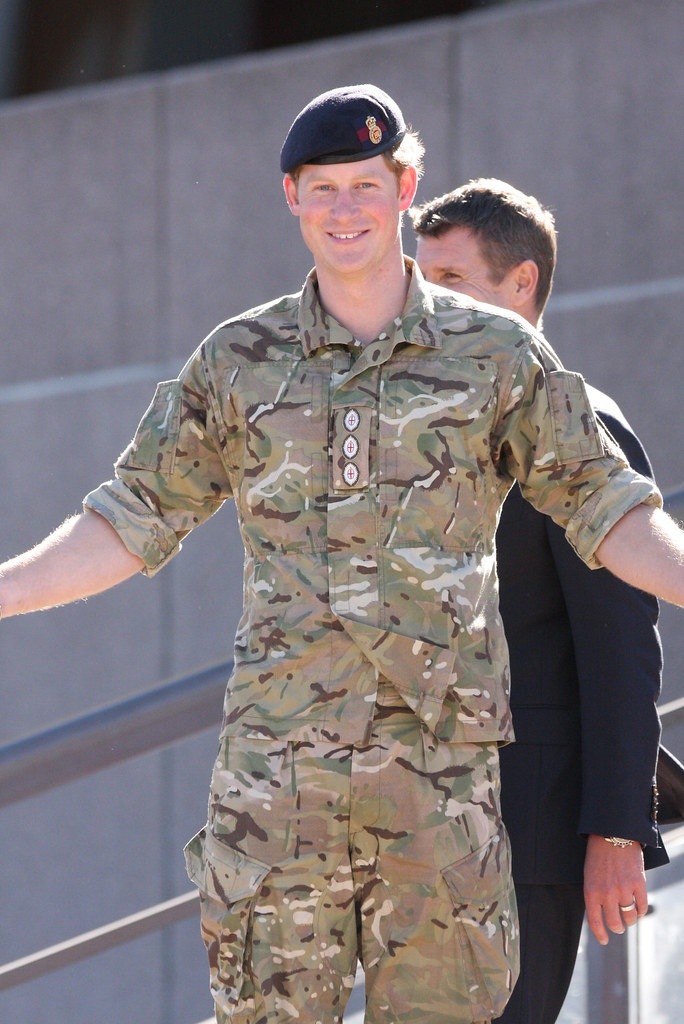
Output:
[279,84,406,173]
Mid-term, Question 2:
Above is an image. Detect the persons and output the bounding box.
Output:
[0,80,681,1024]
[415,177,666,1024]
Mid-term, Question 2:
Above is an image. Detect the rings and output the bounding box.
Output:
[620,901,637,912]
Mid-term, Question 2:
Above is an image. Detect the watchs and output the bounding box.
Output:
[591,835,642,848]
